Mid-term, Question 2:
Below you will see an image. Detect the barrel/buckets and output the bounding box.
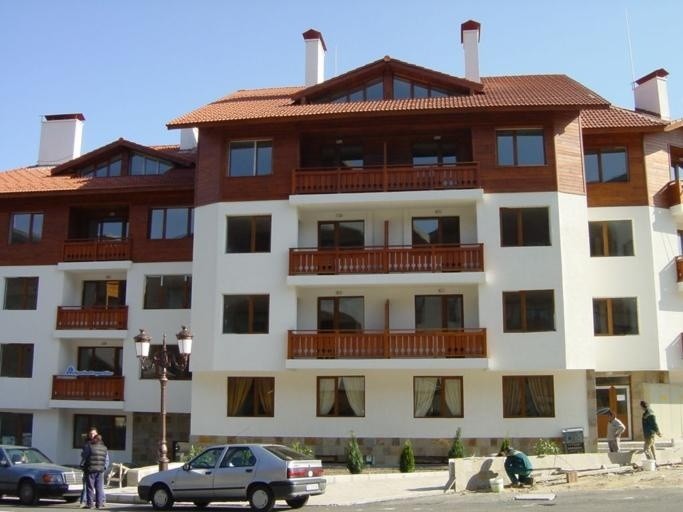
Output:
[489,478,504,492]
[641,460,656,471]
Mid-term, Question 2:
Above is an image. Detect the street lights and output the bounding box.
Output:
[133,325,192,471]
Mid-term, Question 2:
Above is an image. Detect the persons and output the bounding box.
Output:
[503,446,533,488]
[606,410,626,451]
[79,425,109,508]
[639,400,662,467]
[80,434,109,511]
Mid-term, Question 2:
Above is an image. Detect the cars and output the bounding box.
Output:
[137,443,328,512]
[0,445,85,505]
